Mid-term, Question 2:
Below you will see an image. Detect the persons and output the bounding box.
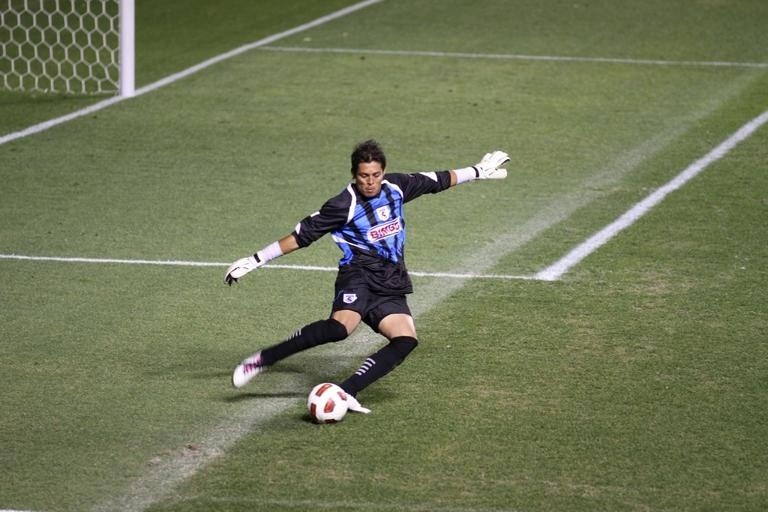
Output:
[222,137,510,414]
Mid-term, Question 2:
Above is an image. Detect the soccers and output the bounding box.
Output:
[306,381,349,425]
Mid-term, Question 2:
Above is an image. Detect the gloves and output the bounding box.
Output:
[223,253,266,287]
[470,151,510,180]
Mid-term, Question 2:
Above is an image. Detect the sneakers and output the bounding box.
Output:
[347,393,371,413]
[232,349,271,390]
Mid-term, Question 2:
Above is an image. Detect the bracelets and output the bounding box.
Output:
[452,165,470,186]
[258,240,283,262]
